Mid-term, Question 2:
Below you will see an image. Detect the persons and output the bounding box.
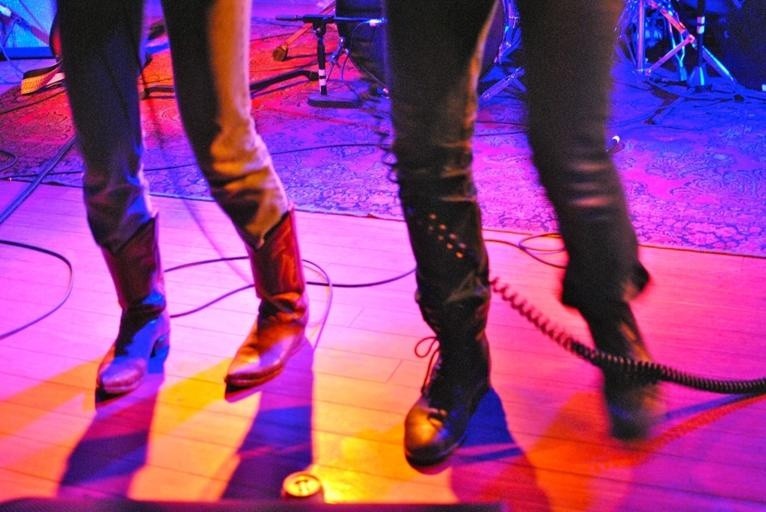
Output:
[52,0,309,399]
[381,0,669,469]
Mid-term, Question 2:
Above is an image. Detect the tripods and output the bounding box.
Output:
[612,0,735,83]
[686,1,714,92]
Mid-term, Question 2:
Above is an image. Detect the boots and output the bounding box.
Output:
[225,202,308,387]
[97,212,169,395]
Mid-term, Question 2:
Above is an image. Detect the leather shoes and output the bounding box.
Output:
[592,316,660,435]
[406,331,490,468]
